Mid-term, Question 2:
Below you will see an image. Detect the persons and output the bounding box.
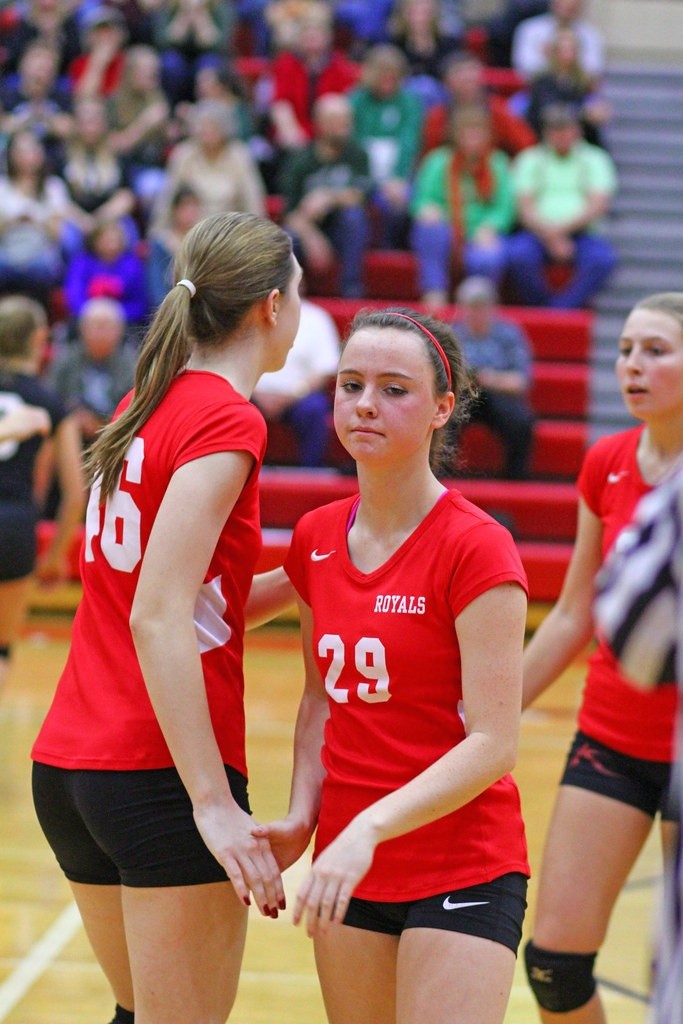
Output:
[0,0,622,657]
[30,210,303,1024]
[523,293,683,1024]
[249,310,527,1024]
[595,461,683,1024]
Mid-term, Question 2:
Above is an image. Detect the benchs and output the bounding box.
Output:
[36,24,591,603]
